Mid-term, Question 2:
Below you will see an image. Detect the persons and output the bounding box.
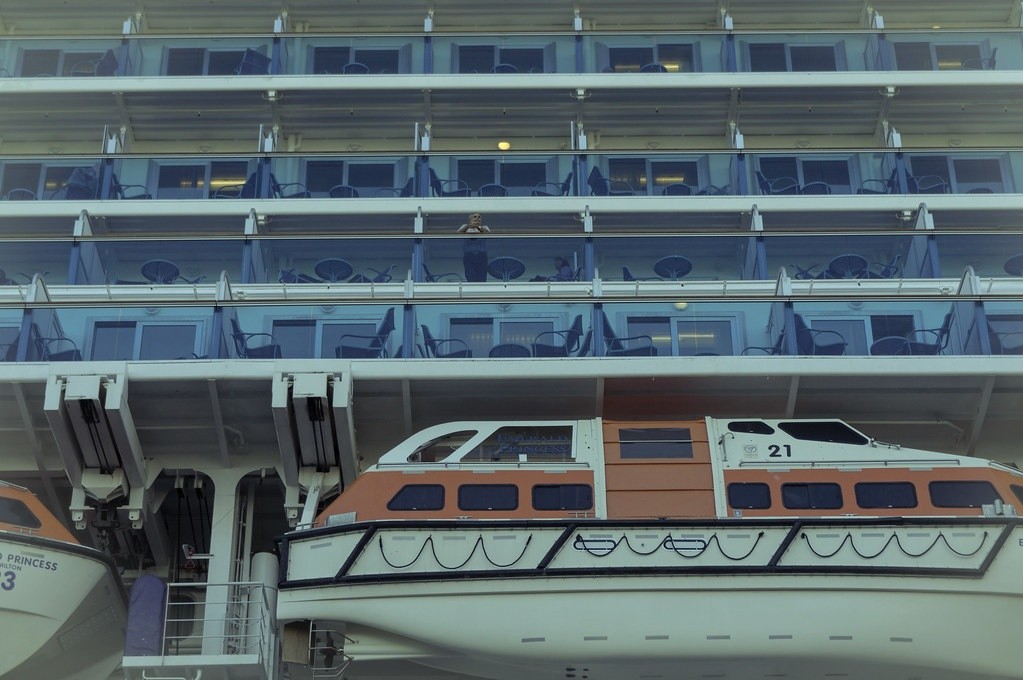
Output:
[529,256,573,282]
[456,213,491,282]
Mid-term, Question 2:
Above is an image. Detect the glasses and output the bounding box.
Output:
[471,216,480,218]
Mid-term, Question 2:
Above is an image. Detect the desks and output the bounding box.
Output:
[829,254,868,279]
[341,61,668,74]
[654,254,693,281]
[870,336,911,355]
[314,257,353,283]
[662,183,693,195]
[7,189,38,200]
[488,255,526,282]
[801,181,831,195]
[328,184,359,199]
[141,259,180,285]
[1004,253,1023,277]
[478,183,509,197]
[489,344,531,357]
[968,188,994,194]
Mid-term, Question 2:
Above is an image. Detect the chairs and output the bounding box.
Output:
[905,167,948,194]
[696,184,731,195]
[429,168,472,197]
[50,167,98,199]
[110,174,152,199]
[269,172,414,198]
[857,255,902,279]
[588,165,637,196]
[230,307,396,357]
[414,313,593,358]
[856,168,897,194]
[960,47,998,70]
[31,322,82,361]
[279,263,399,283]
[422,263,583,282]
[212,171,257,199]
[794,310,952,355]
[756,170,801,195]
[71,47,275,76]
[530,171,573,196]
[622,266,665,281]
[986,319,1023,355]
[742,324,785,355]
[603,311,658,356]
[790,261,836,279]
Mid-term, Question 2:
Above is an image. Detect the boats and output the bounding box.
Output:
[0,0,1023,680]
[274,419,1022,680]
[0,482,128,680]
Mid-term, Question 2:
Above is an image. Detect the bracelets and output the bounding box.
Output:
[478,225,482,230]
